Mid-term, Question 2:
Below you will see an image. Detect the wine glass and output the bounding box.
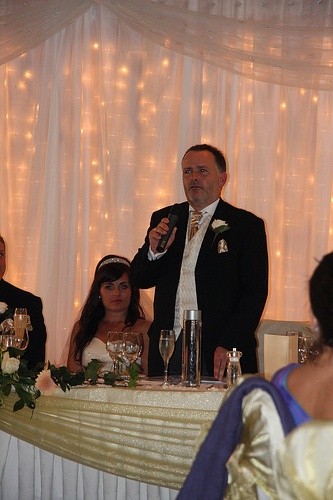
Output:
[158,329,175,386]
[125,332,143,375]
[106,332,125,376]
[0,336,29,359]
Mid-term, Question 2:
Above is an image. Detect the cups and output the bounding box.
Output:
[14,308,27,339]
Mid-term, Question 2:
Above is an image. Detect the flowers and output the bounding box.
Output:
[0,301,117,416]
[209,218,230,249]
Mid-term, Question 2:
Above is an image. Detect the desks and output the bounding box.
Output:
[0,374,238,500]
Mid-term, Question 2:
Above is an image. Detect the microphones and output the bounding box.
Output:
[156,203,183,254]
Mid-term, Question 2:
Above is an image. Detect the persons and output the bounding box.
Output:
[131,145,268,380]
[270,252,333,428]
[67,255,152,375]
[0,235,47,379]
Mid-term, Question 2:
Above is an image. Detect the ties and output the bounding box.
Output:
[189,211,203,239]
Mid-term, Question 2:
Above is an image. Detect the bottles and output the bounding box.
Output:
[226,348,243,388]
[182,310,202,387]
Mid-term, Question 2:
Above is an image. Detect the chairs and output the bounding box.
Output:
[218,320,333,500]
